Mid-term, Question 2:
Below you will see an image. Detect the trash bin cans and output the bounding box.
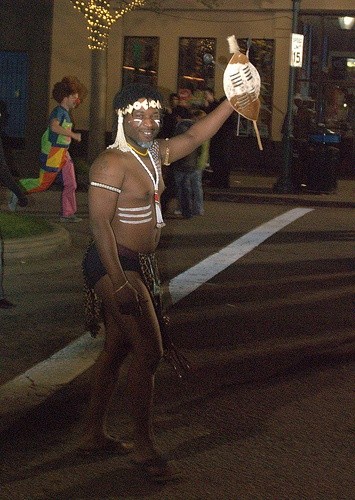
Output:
[299,149,341,194]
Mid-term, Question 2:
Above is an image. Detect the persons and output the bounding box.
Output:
[0,154,28,309]
[158,82,235,219]
[76,83,238,483]
[6,81,83,223]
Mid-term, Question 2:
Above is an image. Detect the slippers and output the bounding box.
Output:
[137,459,183,482]
[75,435,135,454]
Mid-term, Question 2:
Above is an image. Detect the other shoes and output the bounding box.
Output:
[61,216,82,222]
[170,208,193,218]
[8,191,19,212]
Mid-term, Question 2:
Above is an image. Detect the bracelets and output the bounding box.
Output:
[112,281,128,295]
